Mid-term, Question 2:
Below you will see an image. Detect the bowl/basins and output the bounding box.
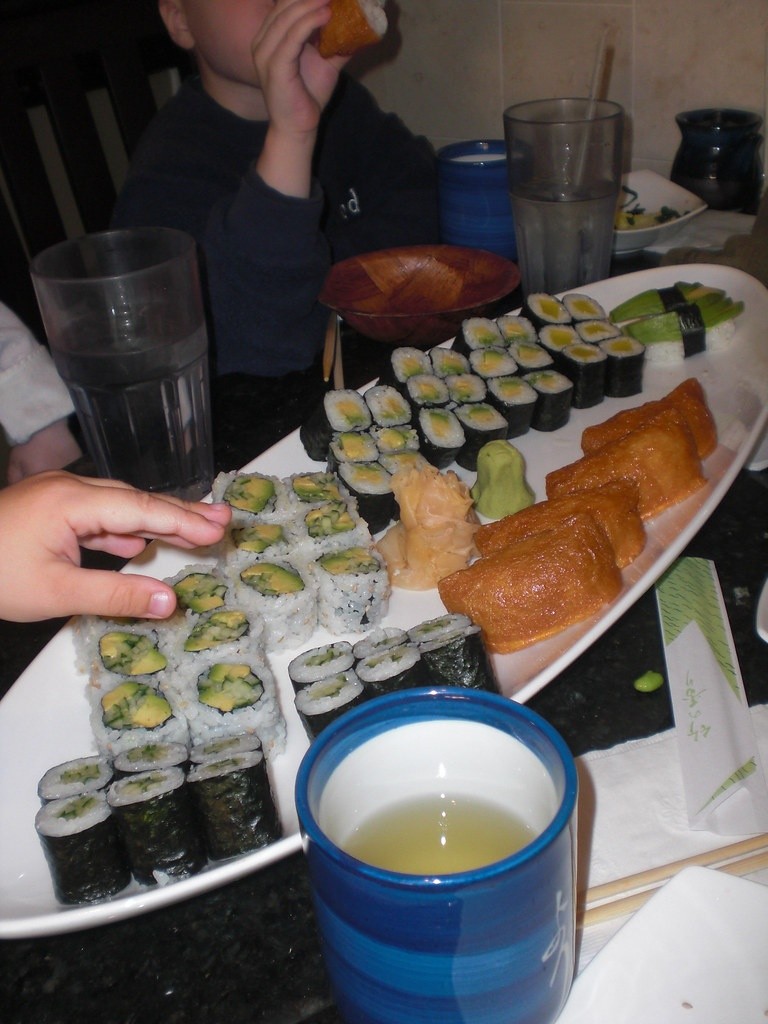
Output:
[610,168,708,256]
[316,244,520,350]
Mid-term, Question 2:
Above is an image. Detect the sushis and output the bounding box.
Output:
[317,0,389,58]
[34,470,501,903]
[299,280,746,657]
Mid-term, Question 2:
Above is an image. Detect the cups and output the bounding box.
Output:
[435,139,534,263]
[502,96,625,303]
[295,687,579,1023]
[30,225,215,504]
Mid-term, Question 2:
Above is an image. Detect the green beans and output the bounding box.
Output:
[634,671,664,691]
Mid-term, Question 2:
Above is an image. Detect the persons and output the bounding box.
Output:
[0,469,232,624]
[0,303,83,484]
[105,0,449,378]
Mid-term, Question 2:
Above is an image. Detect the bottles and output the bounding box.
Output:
[669,107,764,216]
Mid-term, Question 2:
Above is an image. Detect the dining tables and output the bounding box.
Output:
[0,246,768,1024]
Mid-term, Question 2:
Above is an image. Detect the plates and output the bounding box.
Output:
[1,262,768,947]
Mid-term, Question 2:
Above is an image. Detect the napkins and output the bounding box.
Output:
[553,705,768,1024]
[643,210,756,253]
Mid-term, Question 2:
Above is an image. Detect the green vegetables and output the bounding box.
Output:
[618,184,689,225]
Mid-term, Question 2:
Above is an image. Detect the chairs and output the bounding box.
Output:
[0,0,200,346]
